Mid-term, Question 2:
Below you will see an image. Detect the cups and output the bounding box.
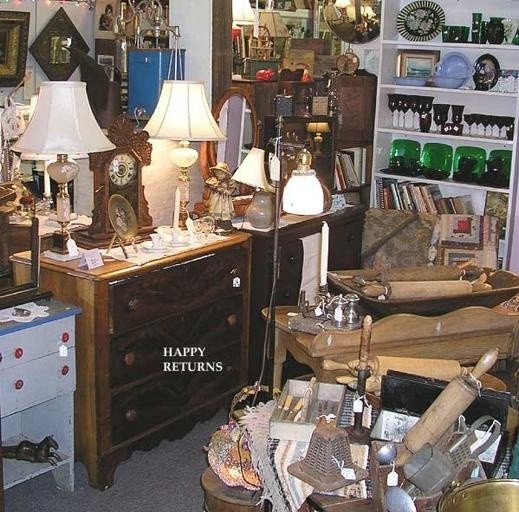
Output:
[442,26,470,43]
[157,226,188,243]
[150,233,165,247]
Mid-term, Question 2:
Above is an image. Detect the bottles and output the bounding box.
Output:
[486,17,506,44]
[245,194,275,229]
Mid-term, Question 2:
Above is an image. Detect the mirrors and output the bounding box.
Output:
[200,86,259,187]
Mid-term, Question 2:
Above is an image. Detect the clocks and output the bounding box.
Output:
[78,115,157,250]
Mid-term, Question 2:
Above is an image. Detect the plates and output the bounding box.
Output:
[108,194,137,240]
[395,1,445,43]
[473,52,500,91]
[435,52,476,86]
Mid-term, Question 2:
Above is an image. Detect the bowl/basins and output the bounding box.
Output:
[392,76,428,86]
[429,75,467,89]
[375,441,397,465]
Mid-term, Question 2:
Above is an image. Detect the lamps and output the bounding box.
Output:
[307,122,331,155]
[231,148,325,228]
[9,81,116,252]
[141,81,228,230]
[232,0,290,38]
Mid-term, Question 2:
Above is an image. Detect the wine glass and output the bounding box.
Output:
[464,113,515,140]
[499,69,519,93]
[192,220,203,245]
[202,220,214,244]
[386,94,465,135]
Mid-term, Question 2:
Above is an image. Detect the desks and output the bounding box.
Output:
[261,306,519,394]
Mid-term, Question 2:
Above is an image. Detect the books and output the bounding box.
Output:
[375,178,474,214]
[332,146,368,191]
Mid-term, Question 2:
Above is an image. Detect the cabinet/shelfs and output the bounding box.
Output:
[369,0,519,270]
[0,204,367,494]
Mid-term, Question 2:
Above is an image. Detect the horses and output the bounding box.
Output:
[4,433,63,466]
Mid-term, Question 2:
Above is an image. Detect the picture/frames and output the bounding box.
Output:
[396,49,440,78]
[0,10,90,88]
